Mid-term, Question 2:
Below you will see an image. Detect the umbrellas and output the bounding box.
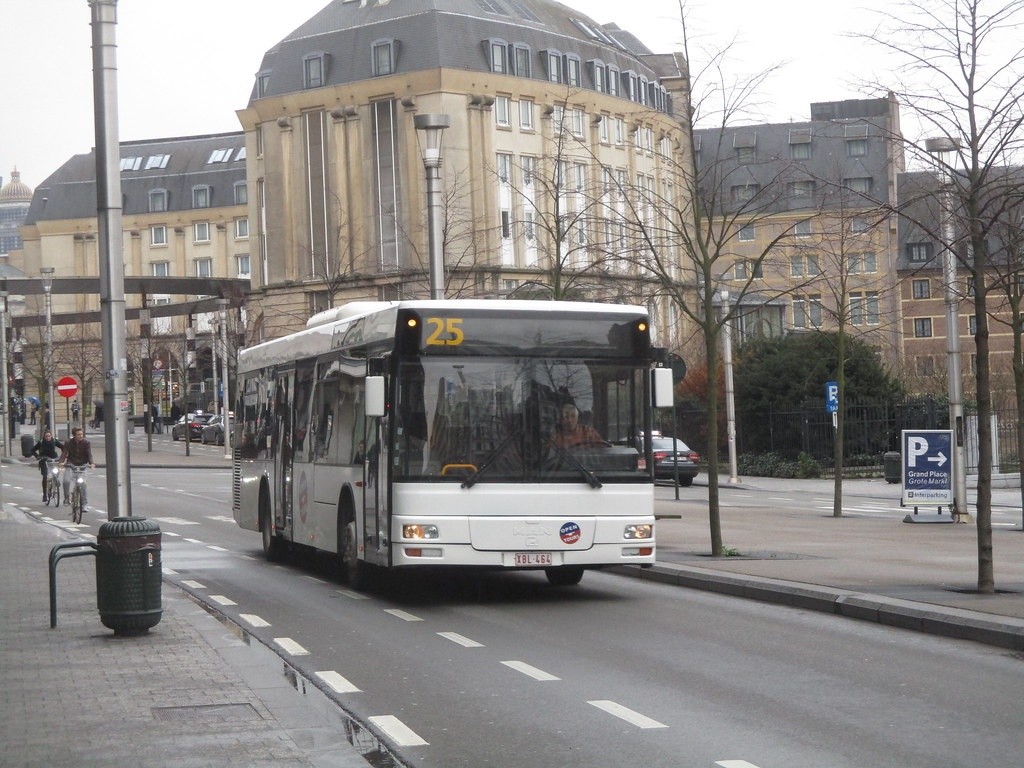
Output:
[26,395,41,409]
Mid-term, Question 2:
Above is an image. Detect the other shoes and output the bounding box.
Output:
[82,504,87,512]
[64,498,69,505]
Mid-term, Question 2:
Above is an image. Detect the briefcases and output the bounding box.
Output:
[43,495,47,501]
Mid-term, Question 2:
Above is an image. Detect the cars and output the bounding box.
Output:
[172,410,213,442]
[200,414,234,447]
[620,430,700,486]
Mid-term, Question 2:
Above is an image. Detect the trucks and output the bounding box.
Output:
[172,381,223,414]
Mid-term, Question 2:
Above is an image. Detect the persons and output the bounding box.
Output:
[545,403,607,456]
[71,400,79,420]
[31,431,64,501]
[28,399,37,425]
[151,403,159,433]
[354,440,365,463]
[59,426,95,512]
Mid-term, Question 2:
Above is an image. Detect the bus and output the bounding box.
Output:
[232,299,674,591]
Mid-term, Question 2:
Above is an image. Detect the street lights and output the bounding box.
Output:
[714,273,741,485]
[40,267,56,438]
[214,299,231,456]
[0,291,11,458]
[412,115,449,299]
[925,137,985,523]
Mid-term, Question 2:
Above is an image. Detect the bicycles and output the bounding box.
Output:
[64,464,92,524]
[41,456,60,507]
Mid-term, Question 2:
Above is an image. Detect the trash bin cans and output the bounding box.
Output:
[884,450,901,483]
[97,515,165,637]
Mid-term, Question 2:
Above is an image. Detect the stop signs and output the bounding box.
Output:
[57,377,78,398]
